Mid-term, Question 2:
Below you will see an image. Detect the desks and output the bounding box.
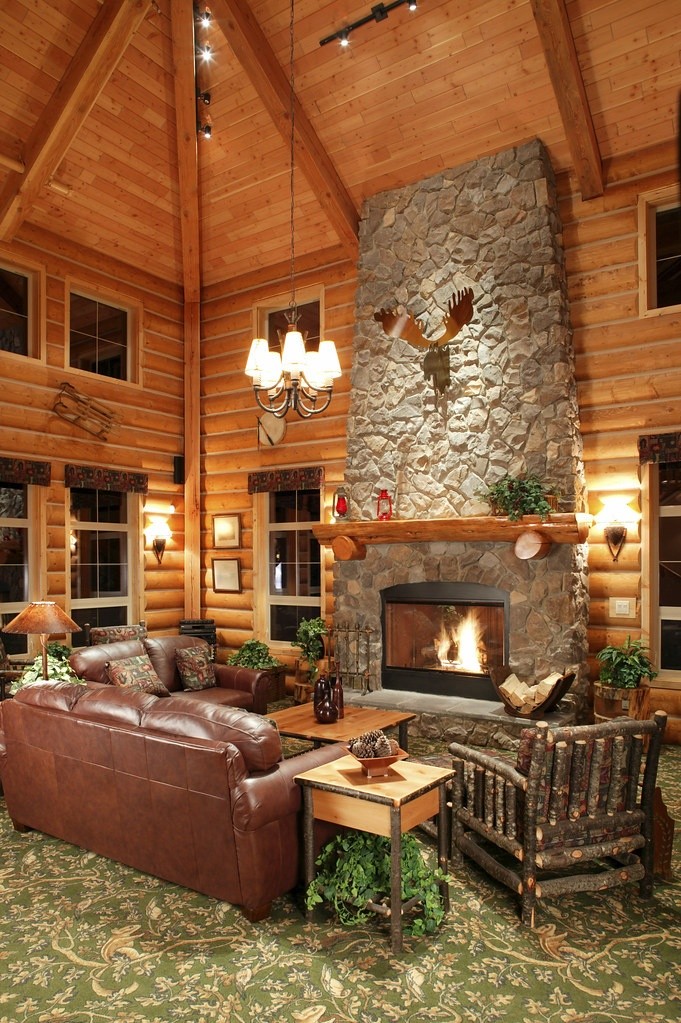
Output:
[293,755,457,952]
[261,701,418,752]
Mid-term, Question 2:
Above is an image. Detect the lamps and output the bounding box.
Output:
[376,488,393,521]
[0,601,83,680]
[332,485,351,522]
[195,87,212,139]
[243,0,342,420]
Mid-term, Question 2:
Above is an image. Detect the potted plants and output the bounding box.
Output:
[596,634,658,689]
[291,616,332,683]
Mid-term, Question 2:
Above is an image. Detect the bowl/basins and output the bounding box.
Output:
[340,744,409,777]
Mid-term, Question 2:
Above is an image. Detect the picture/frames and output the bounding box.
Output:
[211,512,242,549]
[212,557,243,594]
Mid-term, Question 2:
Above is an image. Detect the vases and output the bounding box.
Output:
[314,673,331,715]
[332,661,344,719]
[316,690,338,723]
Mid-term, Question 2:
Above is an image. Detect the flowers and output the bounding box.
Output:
[12,643,88,692]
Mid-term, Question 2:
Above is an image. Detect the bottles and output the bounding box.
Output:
[313,661,344,723]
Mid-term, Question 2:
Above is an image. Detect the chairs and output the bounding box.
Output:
[448,706,668,928]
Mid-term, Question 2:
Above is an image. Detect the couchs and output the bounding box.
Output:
[68,635,280,712]
[0,680,357,924]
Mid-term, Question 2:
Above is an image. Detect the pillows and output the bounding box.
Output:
[175,639,215,692]
[104,652,172,697]
